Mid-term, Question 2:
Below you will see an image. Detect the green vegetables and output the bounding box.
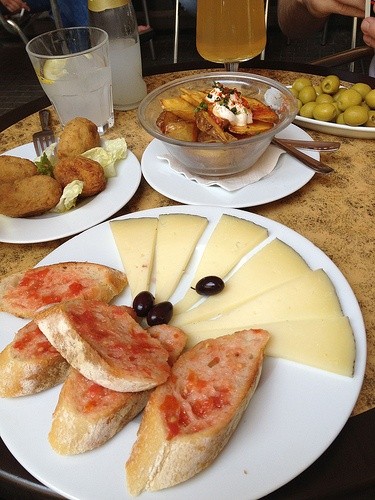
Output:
[193,81,242,115]
[35,152,53,177]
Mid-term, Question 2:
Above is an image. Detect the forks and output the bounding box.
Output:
[32,109,56,157]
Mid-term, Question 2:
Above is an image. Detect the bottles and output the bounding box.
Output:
[87,0,147,110]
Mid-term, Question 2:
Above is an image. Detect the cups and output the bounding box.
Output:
[26,26,115,136]
[0,139,141,244]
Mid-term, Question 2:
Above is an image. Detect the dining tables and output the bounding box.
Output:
[0,59,375,500]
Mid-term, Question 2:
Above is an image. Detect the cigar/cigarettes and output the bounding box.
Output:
[20,3,26,16]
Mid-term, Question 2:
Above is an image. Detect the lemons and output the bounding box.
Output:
[37,57,63,84]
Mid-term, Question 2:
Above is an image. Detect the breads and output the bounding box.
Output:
[0,154,38,183]
[58,118,100,157]
[53,156,105,196]
[0,175,62,217]
[0,261,271,495]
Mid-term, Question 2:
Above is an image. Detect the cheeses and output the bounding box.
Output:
[108,213,356,379]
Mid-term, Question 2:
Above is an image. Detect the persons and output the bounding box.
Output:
[0,0,375,78]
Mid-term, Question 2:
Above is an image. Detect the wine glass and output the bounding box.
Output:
[195,0,269,73]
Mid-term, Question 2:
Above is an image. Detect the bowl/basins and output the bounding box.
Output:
[137,72,298,176]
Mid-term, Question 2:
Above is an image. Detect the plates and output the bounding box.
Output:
[284,85,375,140]
[0,205,367,500]
[141,122,321,207]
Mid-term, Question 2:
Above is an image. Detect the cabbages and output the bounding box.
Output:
[35,143,56,161]
[49,137,128,213]
